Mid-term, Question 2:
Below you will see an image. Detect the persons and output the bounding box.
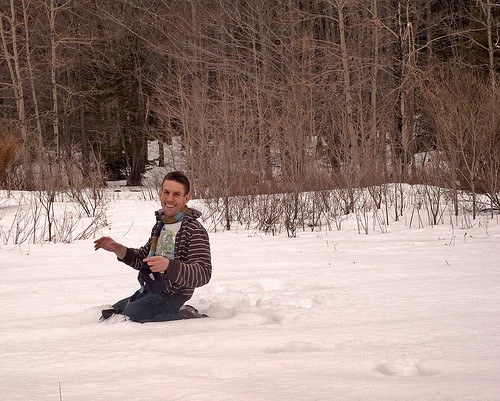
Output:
[93,169,212,323]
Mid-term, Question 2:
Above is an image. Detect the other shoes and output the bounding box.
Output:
[181,305,209,318]
